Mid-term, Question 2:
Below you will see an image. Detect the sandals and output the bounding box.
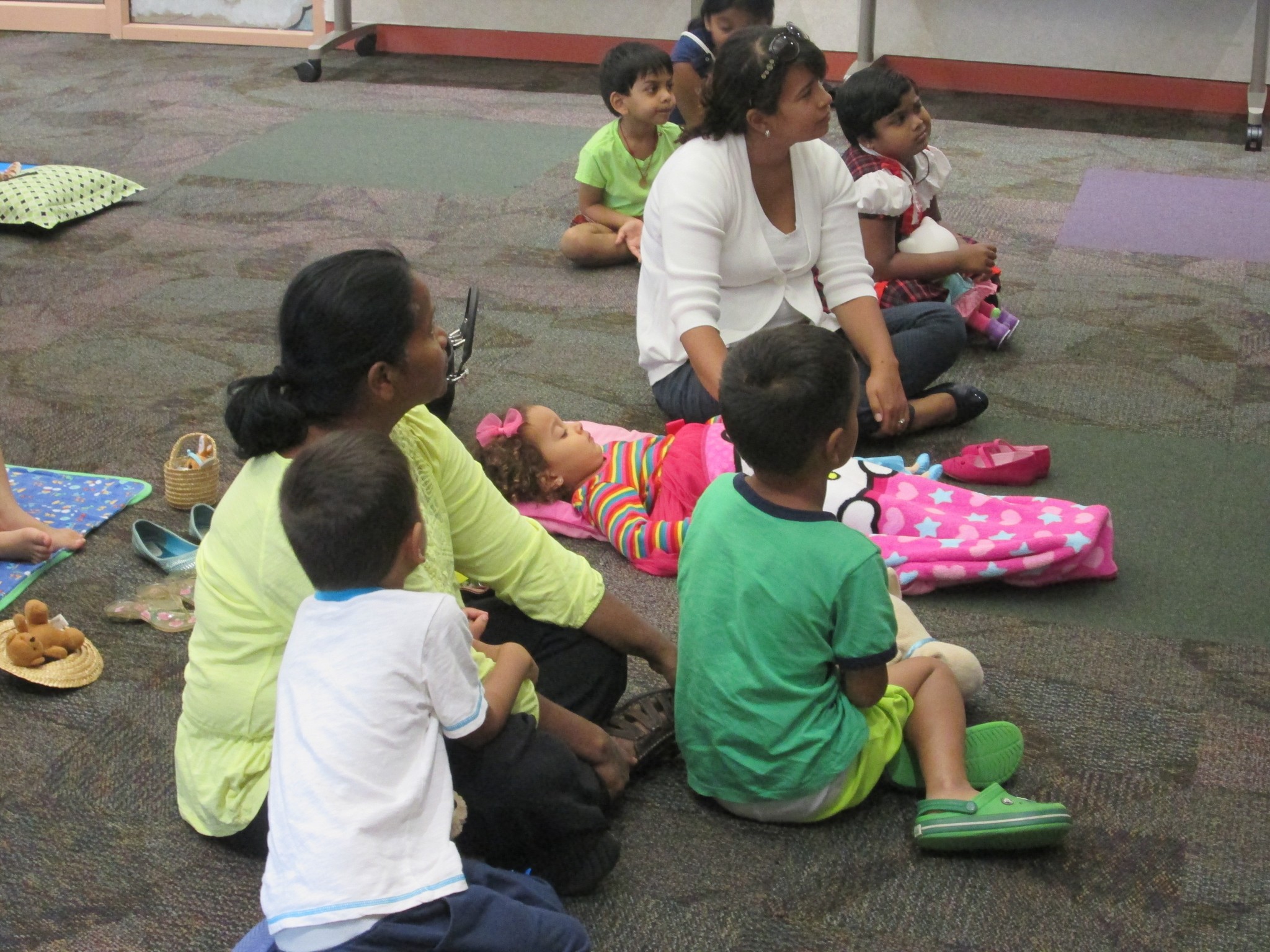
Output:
[912,784,1072,855]
[600,692,686,778]
[887,721,1025,789]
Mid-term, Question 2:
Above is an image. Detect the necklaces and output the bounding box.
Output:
[619,120,659,189]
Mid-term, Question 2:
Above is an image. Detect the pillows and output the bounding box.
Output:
[0,164,148,229]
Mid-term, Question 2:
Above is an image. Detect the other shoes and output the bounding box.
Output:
[130,518,202,575]
[941,447,1037,485]
[961,438,1050,477]
[189,503,217,540]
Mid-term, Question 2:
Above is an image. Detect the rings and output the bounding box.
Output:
[898,419,905,423]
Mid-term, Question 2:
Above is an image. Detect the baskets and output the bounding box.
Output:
[163,431,221,511]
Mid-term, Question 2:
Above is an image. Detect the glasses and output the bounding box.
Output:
[745,20,809,105]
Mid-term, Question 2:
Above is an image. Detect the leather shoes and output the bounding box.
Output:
[908,383,990,426]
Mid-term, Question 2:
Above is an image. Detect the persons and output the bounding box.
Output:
[560,41,692,264]
[216,430,593,952]
[663,0,774,125]
[631,24,988,445]
[836,54,1000,337]
[170,246,676,899]
[0,448,88,565]
[470,403,1121,597]
[672,325,1073,856]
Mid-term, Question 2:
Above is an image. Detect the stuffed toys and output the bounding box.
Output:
[882,567,984,701]
[180,433,214,472]
[898,215,1022,346]
[7,599,85,668]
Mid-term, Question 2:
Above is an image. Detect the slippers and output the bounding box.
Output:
[106,587,197,632]
[135,575,195,607]
